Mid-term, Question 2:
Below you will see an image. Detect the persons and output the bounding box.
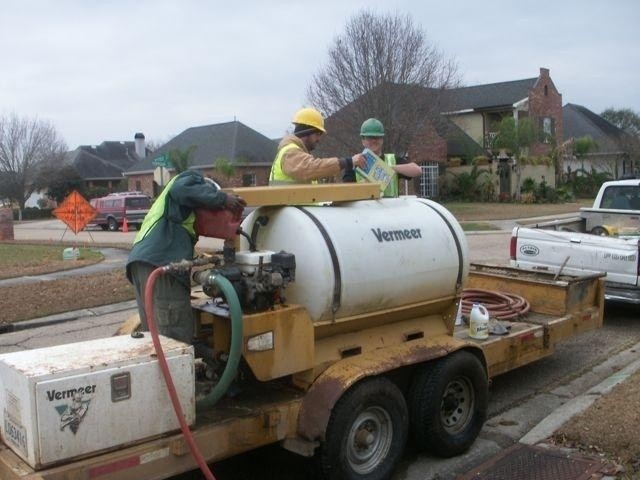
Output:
[266,104,370,186]
[340,116,425,191]
[122,163,249,345]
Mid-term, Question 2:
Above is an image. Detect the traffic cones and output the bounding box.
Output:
[121,216,129,234]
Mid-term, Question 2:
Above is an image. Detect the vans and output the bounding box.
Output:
[88,191,152,232]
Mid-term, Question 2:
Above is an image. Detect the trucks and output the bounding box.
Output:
[0,181,608,480]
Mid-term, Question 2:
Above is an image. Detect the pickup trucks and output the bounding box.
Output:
[509,179,640,307]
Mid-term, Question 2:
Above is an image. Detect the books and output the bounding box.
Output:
[353,147,395,192]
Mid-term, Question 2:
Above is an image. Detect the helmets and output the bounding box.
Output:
[360,118,387,138]
[292,107,328,135]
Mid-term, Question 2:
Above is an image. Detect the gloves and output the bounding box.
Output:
[224,193,249,218]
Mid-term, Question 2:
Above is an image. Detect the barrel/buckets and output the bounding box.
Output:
[63,243,80,259]
[470,302,490,340]
